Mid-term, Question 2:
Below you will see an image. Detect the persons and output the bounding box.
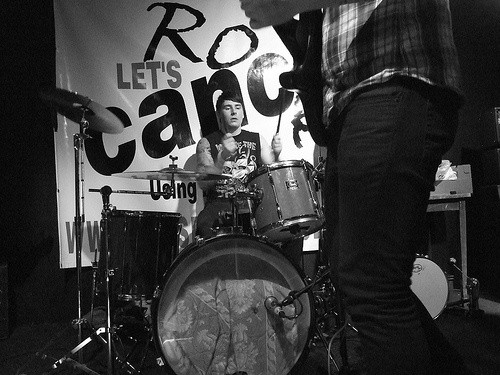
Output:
[240,0,463,375]
[195,91,282,244]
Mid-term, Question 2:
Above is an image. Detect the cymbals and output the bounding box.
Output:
[111,156,233,181]
[43,86,124,134]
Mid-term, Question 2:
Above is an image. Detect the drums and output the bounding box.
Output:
[410,258,480,320]
[153,234,315,375]
[95,209,182,302]
[245,158,326,241]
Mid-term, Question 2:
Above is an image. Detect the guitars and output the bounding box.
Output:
[273,8,328,147]
